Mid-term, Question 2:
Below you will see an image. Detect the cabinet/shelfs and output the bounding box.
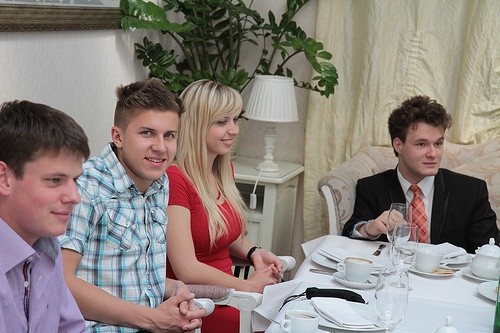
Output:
[231,160,305,284]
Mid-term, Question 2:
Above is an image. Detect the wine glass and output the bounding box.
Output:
[374,266,409,333]
[389,221,418,292]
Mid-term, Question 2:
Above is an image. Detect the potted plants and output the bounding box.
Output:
[119,0,339,161]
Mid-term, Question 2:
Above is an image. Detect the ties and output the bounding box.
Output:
[408,184,428,244]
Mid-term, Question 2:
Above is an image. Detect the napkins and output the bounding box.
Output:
[310,298,375,329]
[438,242,466,259]
[318,246,386,271]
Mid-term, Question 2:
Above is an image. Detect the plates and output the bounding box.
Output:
[461,268,499,301]
[332,272,378,290]
[440,253,472,265]
[293,299,385,332]
[407,266,455,277]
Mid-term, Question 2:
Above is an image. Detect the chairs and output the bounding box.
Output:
[194,251,295,333]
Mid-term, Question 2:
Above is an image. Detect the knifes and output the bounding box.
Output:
[372,245,386,256]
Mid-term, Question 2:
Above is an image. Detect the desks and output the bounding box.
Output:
[261,231,500,333]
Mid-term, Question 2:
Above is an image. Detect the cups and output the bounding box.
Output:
[470,238,500,279]
[387,203,412,246]
[336,257,373,283]
[415,248,450,273]
[280,310,319,333]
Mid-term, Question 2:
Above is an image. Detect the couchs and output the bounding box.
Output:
[317,137,500,250]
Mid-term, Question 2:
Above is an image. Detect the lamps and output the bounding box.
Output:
[244,75,299,173]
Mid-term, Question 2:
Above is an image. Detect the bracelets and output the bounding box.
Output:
[247,246,262,266]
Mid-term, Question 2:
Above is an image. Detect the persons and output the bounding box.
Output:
[0,100,90,333]
[58,77,207,333]
[164,79,285,333]
[342,95,500,255]
